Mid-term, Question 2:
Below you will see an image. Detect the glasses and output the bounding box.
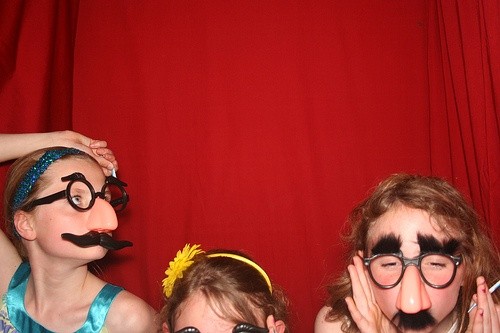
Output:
[22,173,129,212]
[362,252,464,289]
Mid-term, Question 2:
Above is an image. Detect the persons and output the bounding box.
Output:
[153,244,290,333]
[313,173,500,333]
[0,130,159,333]
